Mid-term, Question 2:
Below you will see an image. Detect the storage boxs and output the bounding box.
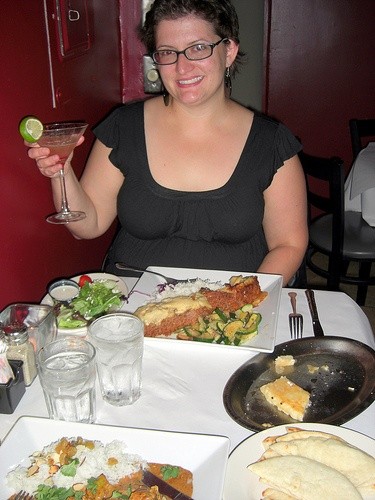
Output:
[0,359,26,414]
[0,304,58,367]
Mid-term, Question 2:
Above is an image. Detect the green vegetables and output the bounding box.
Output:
[33,457,179,500]
[56,278,123,329]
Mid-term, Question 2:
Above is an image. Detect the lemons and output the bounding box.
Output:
[20,115,43,142]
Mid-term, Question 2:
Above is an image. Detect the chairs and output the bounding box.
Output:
[295,118,375,306]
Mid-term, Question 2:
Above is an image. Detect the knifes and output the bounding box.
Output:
[305,290,324,338]
[141,469,194,500]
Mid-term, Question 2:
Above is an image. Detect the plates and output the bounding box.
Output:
[223,422,375,500]
[41,273,128,336]
[0,416,229,500]
[223,337,375,433]
[118,266,284,354]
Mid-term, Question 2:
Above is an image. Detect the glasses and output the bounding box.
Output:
[150,36,224,66]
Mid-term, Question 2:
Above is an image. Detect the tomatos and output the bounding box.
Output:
[79,275,92,286]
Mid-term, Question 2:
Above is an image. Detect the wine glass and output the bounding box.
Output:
[37,122,88,225]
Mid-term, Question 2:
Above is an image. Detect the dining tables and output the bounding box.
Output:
[0,277,375,500]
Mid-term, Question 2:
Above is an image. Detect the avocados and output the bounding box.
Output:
[184,303,262,346]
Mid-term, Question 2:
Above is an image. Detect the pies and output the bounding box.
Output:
[275,355,297,366]
[246,426,375,500]
[259,375,311,421]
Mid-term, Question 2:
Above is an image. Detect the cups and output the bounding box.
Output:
[36,337,97,423]
[49,279,82,304]
[88,312,145,406]
[0,303,57,371]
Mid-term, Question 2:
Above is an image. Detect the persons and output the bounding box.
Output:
[23,0,310,288]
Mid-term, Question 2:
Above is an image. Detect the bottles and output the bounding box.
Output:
[0,322,39,386]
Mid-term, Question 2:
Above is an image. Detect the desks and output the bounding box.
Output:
[345,141,375,227]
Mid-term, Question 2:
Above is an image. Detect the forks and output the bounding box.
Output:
[14,489,35,500]
[288,292,306,341]
[116,263,198,284]
